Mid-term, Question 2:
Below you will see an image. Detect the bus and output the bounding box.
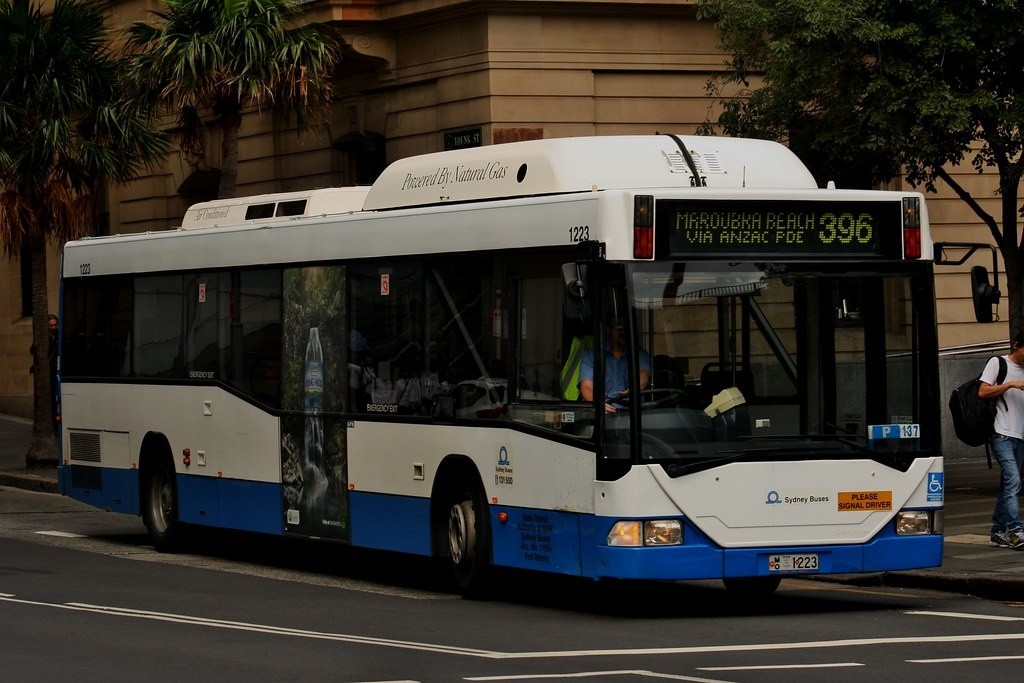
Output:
[58,134,1001,599]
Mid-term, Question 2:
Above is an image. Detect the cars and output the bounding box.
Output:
[445,379,508,419]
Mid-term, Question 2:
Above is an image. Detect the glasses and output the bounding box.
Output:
[609,323,624,331]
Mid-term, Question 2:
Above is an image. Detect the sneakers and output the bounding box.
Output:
[988,531,1011,547]
[1008,529,1024,549]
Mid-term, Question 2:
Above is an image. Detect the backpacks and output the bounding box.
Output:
[947,354,1009,447]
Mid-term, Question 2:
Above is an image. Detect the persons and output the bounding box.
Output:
[577,323,651,437]
[978,330,1024,548]
[29,315,60,436]
